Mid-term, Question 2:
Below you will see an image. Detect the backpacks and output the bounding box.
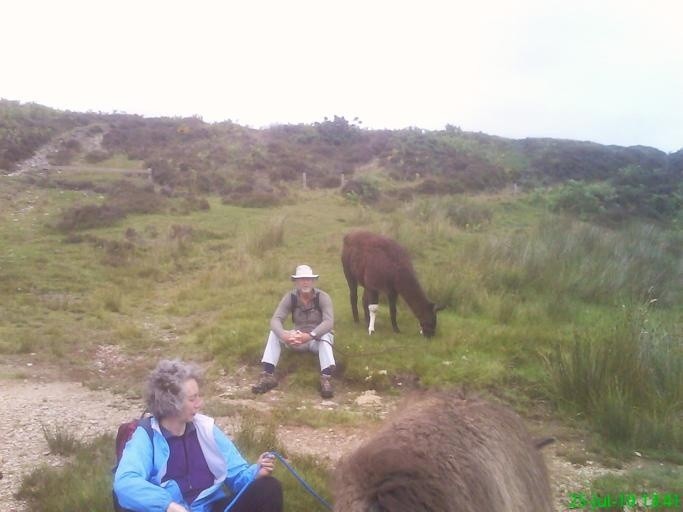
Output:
[115,422,137,468]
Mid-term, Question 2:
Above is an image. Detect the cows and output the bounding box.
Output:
[330,386,559,511]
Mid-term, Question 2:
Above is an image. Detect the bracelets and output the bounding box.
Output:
[309,331,315,338]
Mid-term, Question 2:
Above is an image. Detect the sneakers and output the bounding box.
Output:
[319,374,333,397]
[252,372,277,392]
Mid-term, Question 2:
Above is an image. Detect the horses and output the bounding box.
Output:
[342,226,446,340]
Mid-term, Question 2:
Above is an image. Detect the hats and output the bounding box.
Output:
[290,265,319,278]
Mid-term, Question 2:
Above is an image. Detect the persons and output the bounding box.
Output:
[249,263,339,399]
[109,358,284,511]
